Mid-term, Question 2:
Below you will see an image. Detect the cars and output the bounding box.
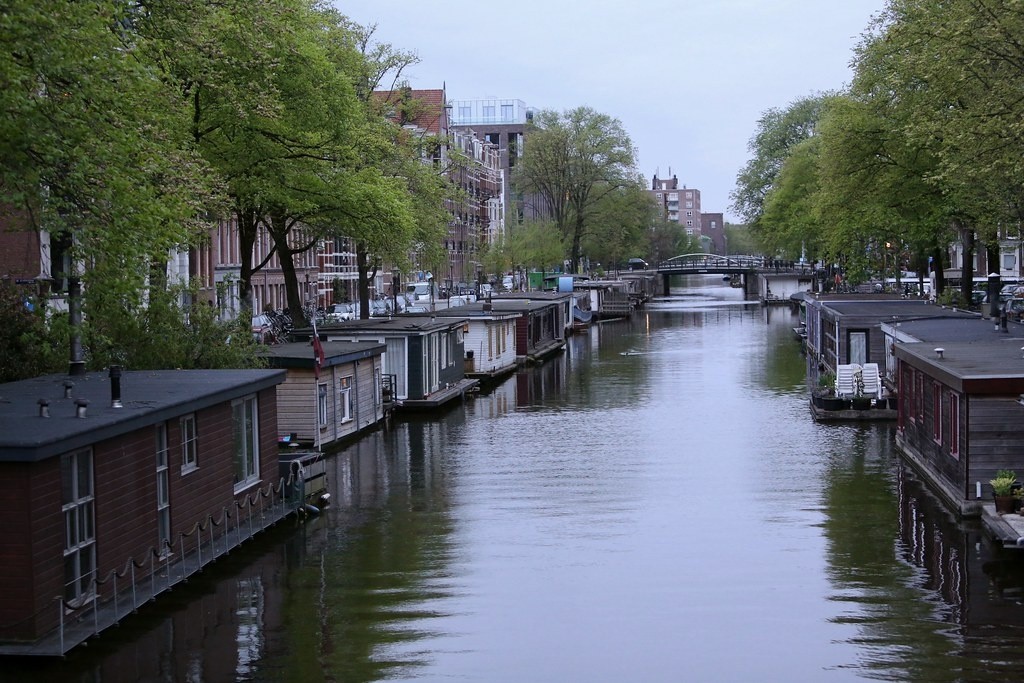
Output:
[501,272,523,290]
[327,292,464,324]
[628,257,647,271]
[971,284,1024,324]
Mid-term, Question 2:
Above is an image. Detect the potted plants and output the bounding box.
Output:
[992,467,1024,516]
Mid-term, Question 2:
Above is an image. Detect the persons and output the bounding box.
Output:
[277,304,325,317]
[763,255,795,268]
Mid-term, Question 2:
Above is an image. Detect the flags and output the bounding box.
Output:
[312,326,324,382]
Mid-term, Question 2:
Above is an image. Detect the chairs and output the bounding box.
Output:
[833,361,884,399]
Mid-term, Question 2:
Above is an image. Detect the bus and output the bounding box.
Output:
[932,267,962,303]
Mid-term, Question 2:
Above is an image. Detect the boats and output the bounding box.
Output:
[723,274,731,281]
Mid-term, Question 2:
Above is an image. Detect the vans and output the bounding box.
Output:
[405,281,439,301]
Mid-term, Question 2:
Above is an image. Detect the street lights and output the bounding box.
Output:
[445,277,453,307]
[476,262,484,300]
[304,265,311,307]
[389,266,401,314]
